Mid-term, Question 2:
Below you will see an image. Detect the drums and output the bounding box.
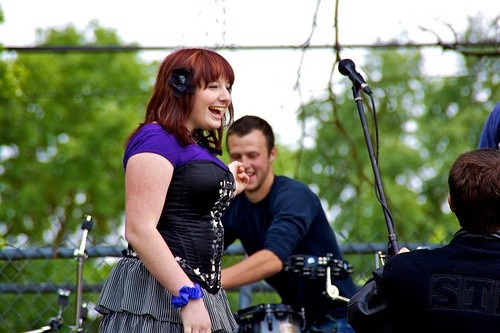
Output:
[237,303,306,333]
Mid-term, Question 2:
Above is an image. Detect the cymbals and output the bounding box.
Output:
[282,255,354,280]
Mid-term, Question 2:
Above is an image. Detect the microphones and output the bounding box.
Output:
[338,59,372,95]
